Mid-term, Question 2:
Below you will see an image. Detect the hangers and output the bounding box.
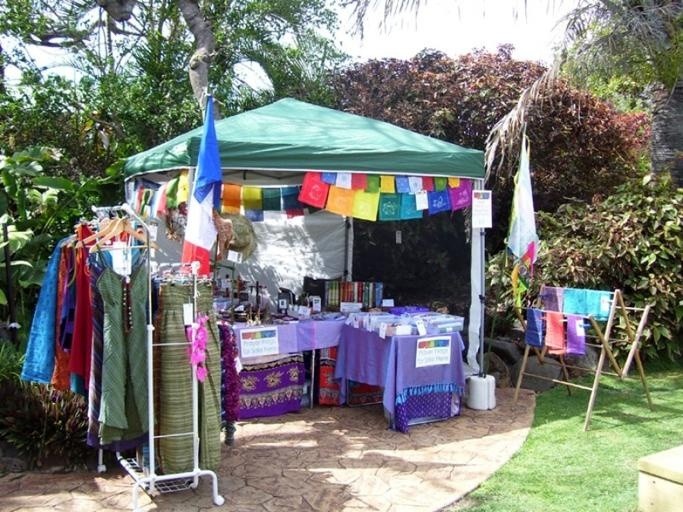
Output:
[60,206,159,254]
[149,263,210,288]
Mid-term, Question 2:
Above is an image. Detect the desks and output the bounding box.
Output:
[216,304,466,428]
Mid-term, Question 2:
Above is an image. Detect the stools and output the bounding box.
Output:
[635,442,683,511]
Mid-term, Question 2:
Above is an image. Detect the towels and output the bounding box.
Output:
[524,285,612,356]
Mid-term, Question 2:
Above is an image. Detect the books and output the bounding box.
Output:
[303,277,384,311]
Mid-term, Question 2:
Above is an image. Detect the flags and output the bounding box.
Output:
[184,96,223,280]
[508,133,540,262]
